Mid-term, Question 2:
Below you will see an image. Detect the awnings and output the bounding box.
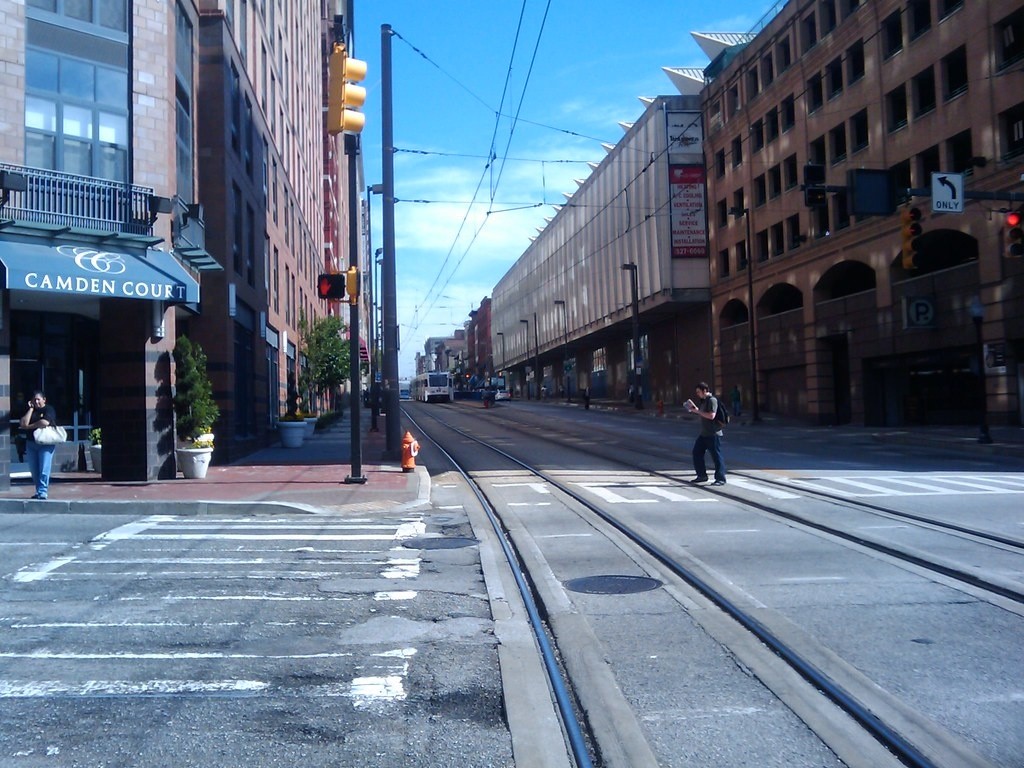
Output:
[0,241,200,303]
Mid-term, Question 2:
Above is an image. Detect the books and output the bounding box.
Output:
[684,399,698,412]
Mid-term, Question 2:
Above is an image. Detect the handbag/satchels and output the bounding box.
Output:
[33,415,67,445]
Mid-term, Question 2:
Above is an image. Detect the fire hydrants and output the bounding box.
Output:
[400,431,421,473]
[657,400,665,417]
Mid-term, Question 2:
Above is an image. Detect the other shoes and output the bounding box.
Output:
[691,477,707,482]
[712,481,724,485]
[31,494,41,498]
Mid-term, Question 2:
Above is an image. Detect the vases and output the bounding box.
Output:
[176,449,213,479]
[278,422,307,449]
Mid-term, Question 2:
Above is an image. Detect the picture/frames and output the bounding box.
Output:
[303,418,318,438]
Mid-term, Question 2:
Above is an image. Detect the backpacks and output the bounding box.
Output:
[703,396,730,431]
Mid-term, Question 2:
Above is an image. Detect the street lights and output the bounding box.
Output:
[367,183,384,432]
[374,248,384,415]
[554,300,572,403]
[620,262,640,411]
[728,206,761,422]
[520,319,531,401]
[497,333,505,370]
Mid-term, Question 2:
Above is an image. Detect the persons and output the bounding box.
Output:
[583,384,592,410]
[19,391,55,500]
[627,384,635,403]
[690,381,727,486]
[558,384,565,398]
[542,386,549,400]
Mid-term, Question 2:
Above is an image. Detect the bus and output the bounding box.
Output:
[409,371,455,403]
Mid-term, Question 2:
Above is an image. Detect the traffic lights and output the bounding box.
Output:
[326,42,367,136]
[317,273,345,299]
[1002,210,1024,261]
[900,204,924,272]
[803,163,828,207]
[845,167,896,218]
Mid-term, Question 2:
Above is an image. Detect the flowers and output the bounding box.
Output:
[279,369,302,422]
[172,334,213,449]
[298,376,316,418]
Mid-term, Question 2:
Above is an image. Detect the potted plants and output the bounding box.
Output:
[89,428,101,473]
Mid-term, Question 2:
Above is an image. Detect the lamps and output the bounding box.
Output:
[0,171,26,210]
[178,203,204,230]
[145,196,172,227]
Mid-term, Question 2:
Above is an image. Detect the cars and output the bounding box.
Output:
[481,385,511,402]
